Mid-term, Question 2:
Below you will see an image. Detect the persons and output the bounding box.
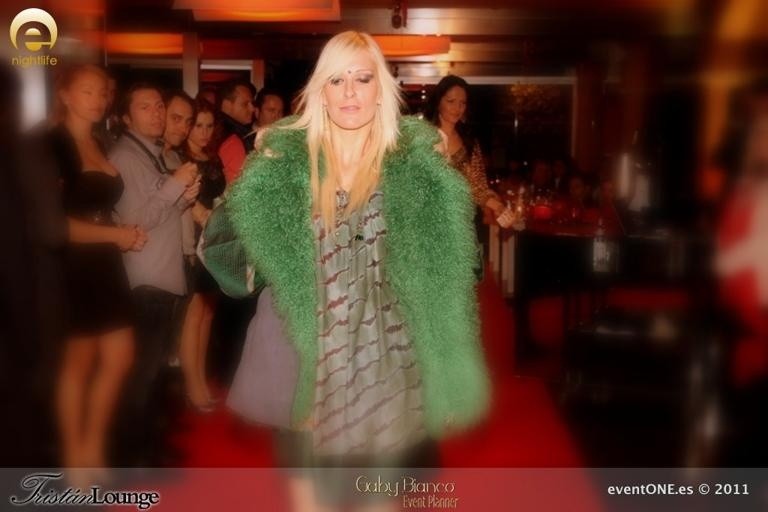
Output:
[22,29,767,511]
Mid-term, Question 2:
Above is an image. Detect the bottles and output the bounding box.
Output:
[503,186,527,233]
